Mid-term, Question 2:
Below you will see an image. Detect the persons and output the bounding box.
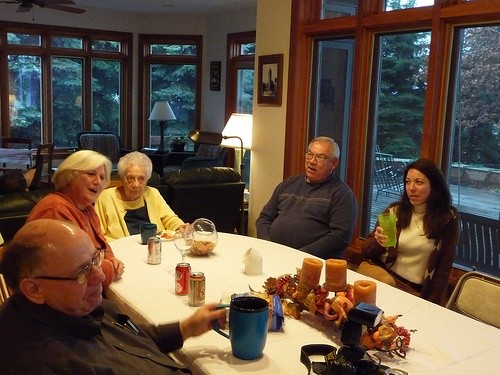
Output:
[0,219,226,375]
[356,158,461,304]
[93,151,190,243]
[255,136,358,259]
[25,150,125,286]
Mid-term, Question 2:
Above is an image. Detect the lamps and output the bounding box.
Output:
[189,130,245,205]
[220,112,252,205]
[148,101,177,154]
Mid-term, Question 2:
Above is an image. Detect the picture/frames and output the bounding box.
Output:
[257,53,284,107]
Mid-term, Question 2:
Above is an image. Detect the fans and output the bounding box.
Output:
[0,0,86,21]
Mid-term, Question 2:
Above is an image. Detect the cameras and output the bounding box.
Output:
[325,301,409,375]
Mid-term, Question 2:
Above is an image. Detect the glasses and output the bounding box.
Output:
[125,174,146,185]
[303,150,338,162]
[32,248,105,286]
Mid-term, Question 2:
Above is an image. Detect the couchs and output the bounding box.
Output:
[154,144,235,171]
[0,166,245,238]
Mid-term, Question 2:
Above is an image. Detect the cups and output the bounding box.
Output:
[378,213,400,247]
[140,224,157,245]
[212,296,270,361]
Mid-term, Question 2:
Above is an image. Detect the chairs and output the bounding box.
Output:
[446,270,500,329]
[76,131,135,170]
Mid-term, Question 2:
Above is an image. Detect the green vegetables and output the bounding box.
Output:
[157,230,164,235]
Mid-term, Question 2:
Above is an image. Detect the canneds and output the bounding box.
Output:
[175,263,192,296]
[188,272,206,306]
[147,237,161,265]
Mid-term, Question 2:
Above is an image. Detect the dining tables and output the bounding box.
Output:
[101,229,500,375]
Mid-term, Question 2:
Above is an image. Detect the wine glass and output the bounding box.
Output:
[173,229,195,262]
